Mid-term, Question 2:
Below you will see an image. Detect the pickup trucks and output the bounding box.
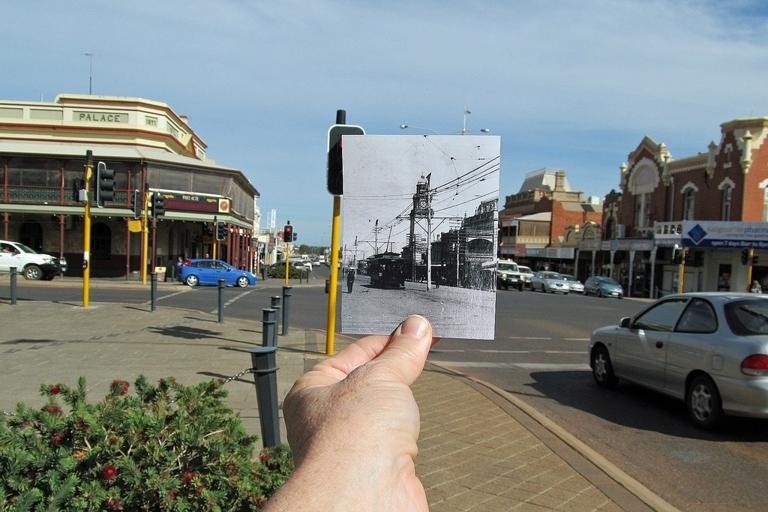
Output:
[0,240,66,282]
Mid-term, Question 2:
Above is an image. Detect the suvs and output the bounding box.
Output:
[496,257,524,292]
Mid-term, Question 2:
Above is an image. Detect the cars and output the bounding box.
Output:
[589,290,768,426]
[534,271,570,295]
[518,265,534,289]
[177,259,259,290]
[585,275,624,300]
[561,274,585,295]
[288,253,326,272]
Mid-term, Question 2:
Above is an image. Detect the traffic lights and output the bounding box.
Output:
[152,195,166,219]
[131,189,140,219]
[285,227,291,242]
[751,254,759,266]
[741,250,748,265]
[674,249,683,264]
[293,233,297,241]
[97,162,117,207]
[217,223,224,240]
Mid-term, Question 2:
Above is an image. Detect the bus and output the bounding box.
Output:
[356,259,368,276]
[366,252,407,290]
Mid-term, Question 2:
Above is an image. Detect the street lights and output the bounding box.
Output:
[84,52,95,96]
[401,102,490,136]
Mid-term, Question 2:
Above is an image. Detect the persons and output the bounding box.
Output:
[347,270,354,293]
[256,314,440,511]
[751,280,762,293]
[3,245,11,252]
